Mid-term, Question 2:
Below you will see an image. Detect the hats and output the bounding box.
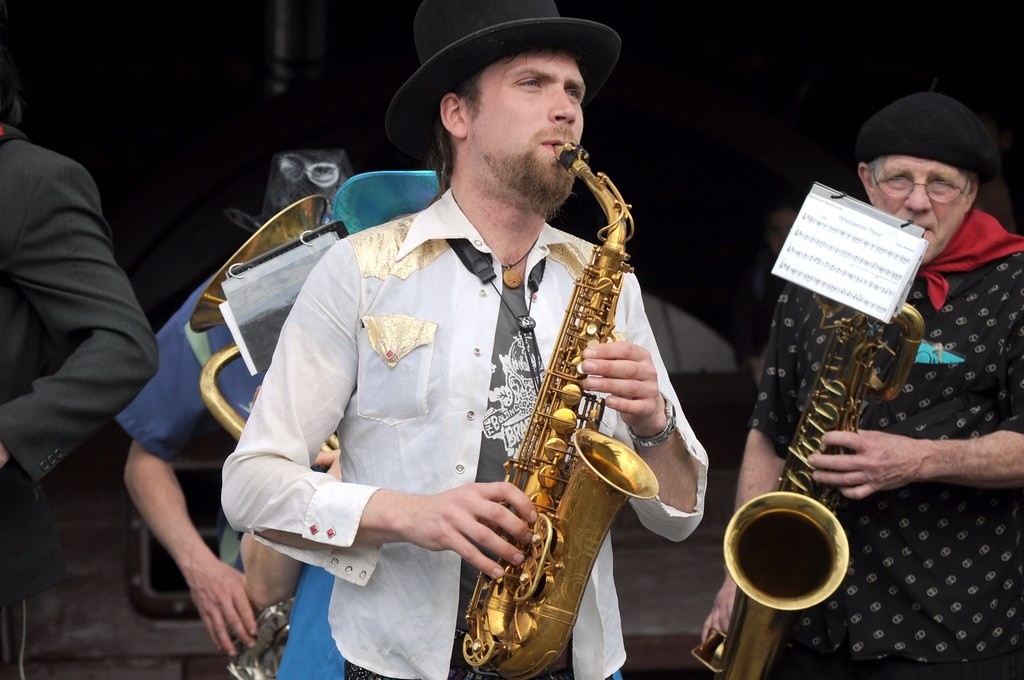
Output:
[385,0,622,158]
[858,91,1005,178]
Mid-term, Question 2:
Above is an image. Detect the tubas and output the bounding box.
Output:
[188,194,358,680]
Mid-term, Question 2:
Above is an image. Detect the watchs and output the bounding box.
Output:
[629,391,678,448]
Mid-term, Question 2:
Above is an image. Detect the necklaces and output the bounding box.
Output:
[452,189,540,289]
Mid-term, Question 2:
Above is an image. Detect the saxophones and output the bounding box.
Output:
[461,143,659,680]
[690,293,923,680]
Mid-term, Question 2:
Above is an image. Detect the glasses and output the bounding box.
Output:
[871,169,973,202]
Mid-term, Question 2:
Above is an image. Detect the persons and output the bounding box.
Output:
[0,92,1023,679]
[219,1,709,680]
